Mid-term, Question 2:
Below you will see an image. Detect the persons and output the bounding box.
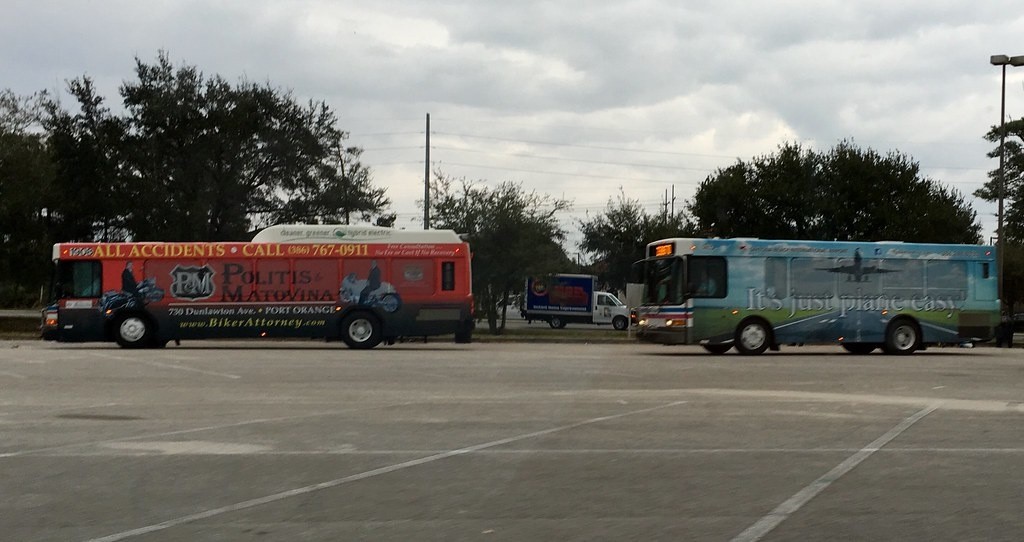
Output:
[81,273,98,296]
[698,271,720,296]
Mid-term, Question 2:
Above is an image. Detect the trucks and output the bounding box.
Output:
[520,272,631,331]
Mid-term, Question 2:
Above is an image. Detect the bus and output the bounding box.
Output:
[635,235,1003,356]
[43,224,477,351]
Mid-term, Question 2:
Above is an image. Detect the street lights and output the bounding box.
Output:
[990,54,1024,303]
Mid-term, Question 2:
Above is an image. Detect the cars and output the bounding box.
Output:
[1009,313,1024,333]
[630,307,635,323]
